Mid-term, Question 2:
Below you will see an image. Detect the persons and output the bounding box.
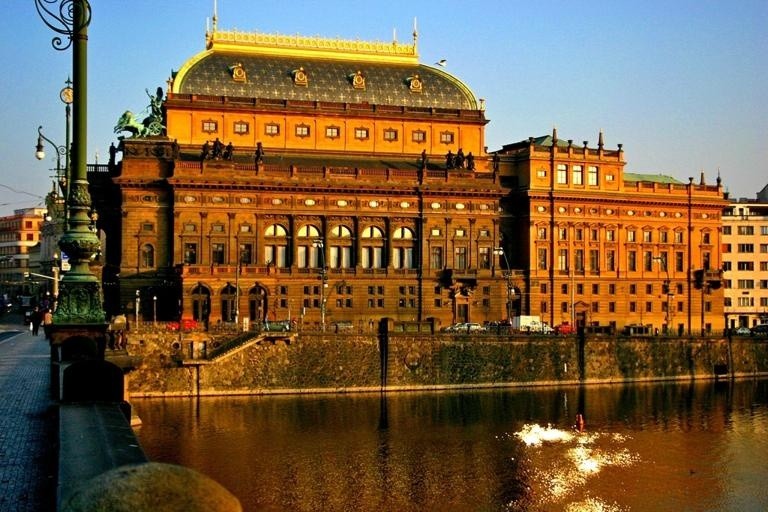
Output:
[492,151,501,171]
[201,140,210,159]
[255,142,265,161]
[446,147,476,170]
[211,136,222,159]
[421,149,427,169]
[28,304,53,340]
[172,138,181,160]
[225,141,234,160]
[145,86,164,122]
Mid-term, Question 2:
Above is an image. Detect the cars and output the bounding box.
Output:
[444,322,482,332]
[736,327,751,334]
[166,319,198,330]
[264,321,289,332]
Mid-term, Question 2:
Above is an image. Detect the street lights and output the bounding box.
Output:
[493,247,515,317]
[653,256,675,336]
[36,126,60,204]
[313,240,328,332]
